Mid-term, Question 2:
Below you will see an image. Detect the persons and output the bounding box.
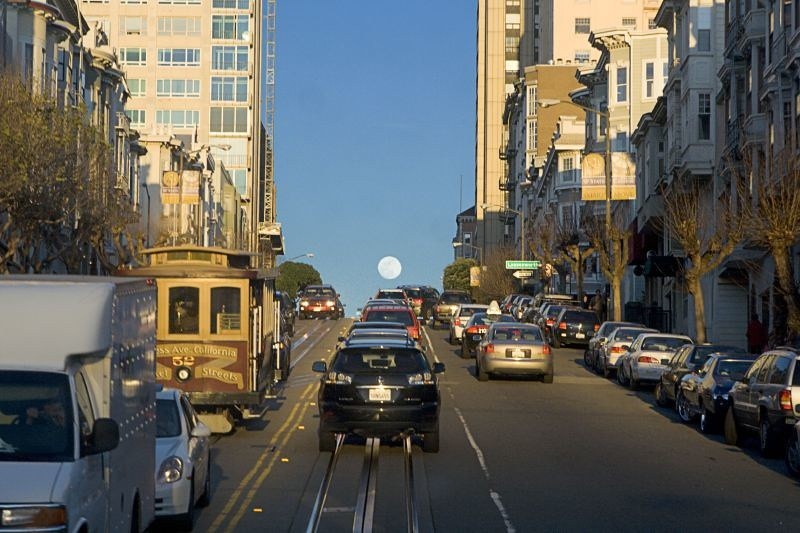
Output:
[747,314,800,355]
[589,289,604,325]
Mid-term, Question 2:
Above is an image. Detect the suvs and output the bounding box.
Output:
[722,344,800,461]
[311,335,446,454]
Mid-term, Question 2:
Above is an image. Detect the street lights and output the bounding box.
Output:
[175,142,233,247]
[452,242,483,305]
[536,98,614,321]
[479,203,525,293]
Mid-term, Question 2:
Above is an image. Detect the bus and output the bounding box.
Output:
[108,243,295,438]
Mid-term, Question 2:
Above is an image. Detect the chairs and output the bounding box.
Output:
[387,353,419,374]
[340,351,370,370]
[495,333,508,340]
[524,333,538,341]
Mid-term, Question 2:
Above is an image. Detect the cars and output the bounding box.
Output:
[616,332,696,392]
[152,386,213,533]
[654,342,745,416]
[675,351,762,436]
[296,283,441,372]
[594,325,668,379]
[446,304,504,346]
[471,321,554,384]
[497,291,602,350]
[430,288,475,330]
[583,320,649,375]
[459,313,521,360]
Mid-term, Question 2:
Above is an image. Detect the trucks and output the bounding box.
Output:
[0,271,160,533]
